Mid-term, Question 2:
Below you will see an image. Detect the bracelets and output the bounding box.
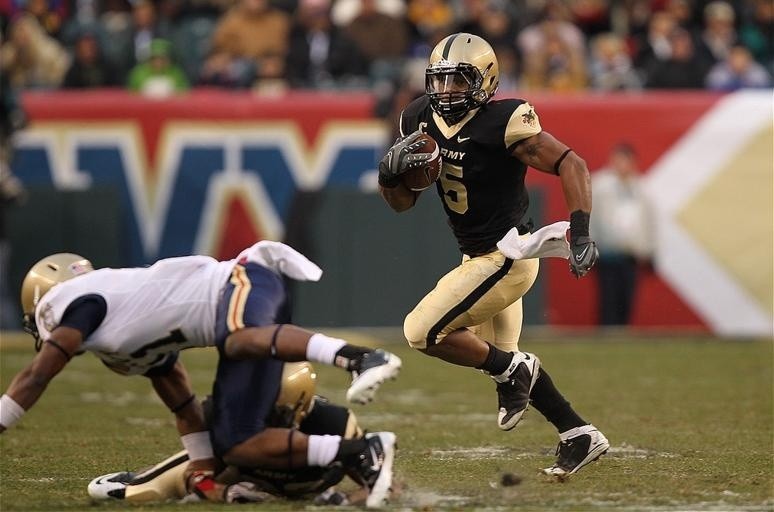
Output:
[181,430,216,464]
[0,394,30,432]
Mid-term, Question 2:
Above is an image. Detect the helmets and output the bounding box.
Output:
[425,33,499,117]
[275,361,317,430]
[20,252,94,322]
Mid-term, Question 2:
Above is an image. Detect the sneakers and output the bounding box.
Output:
[346,348,403,405]
[538,423,611,479]
[490,351,541,431]
[87,471,140,501]
[357,431,397,512]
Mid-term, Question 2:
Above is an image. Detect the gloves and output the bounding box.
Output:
[569,235,599,280]
[378,130,432,188]
[224,481,272,504]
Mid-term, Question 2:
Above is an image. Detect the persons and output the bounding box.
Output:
[0,241,404,511]
[86,357,406,508]
[589,143,654,327]
[0,0,774,96]
[379,32,613,482]
[1,66,30,326]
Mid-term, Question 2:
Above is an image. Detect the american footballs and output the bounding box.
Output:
[403,134,442,192]
